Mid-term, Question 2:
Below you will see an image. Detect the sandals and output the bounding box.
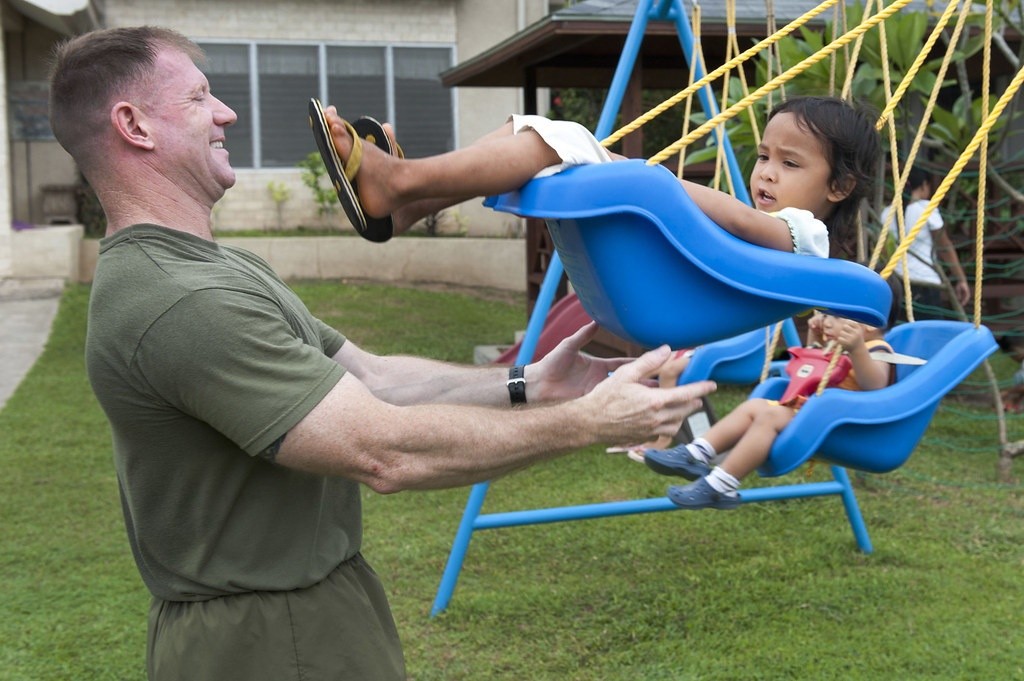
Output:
[643,443,709,480]
[667,477,740,510]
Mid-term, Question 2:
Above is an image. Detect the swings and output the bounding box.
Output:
[481,0,1024,487]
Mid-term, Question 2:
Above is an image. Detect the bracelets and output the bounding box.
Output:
[506,360,528,411]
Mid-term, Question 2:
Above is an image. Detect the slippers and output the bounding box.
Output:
[308,98,369,233]
[352,116,406,243]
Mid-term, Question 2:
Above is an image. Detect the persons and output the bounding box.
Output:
[879,167,973,325]
[49,24,717,680]
[306,95,881,260]
[645,306,897,510]
